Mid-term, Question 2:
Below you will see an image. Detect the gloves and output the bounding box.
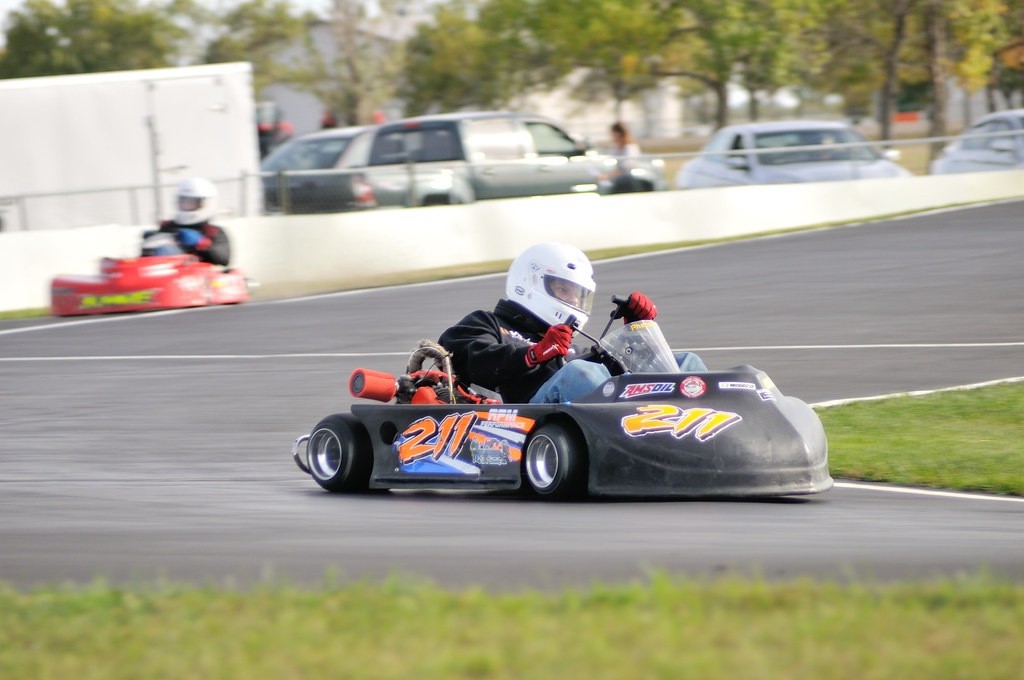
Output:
[179,228,213,250]
[610,292,657,325]
[523,323,572,368]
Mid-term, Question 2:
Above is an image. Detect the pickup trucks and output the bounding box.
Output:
[261,113,663,213]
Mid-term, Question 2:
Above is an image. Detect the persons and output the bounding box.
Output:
[611,123,640,171]
[141,176,231,266]
[434,242,656,404]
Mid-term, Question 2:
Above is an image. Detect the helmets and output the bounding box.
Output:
[173,177,219,225]
[505,243,595,338]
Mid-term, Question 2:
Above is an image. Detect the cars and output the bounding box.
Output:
[923,107,1023,175]
[677,120,915,187]
